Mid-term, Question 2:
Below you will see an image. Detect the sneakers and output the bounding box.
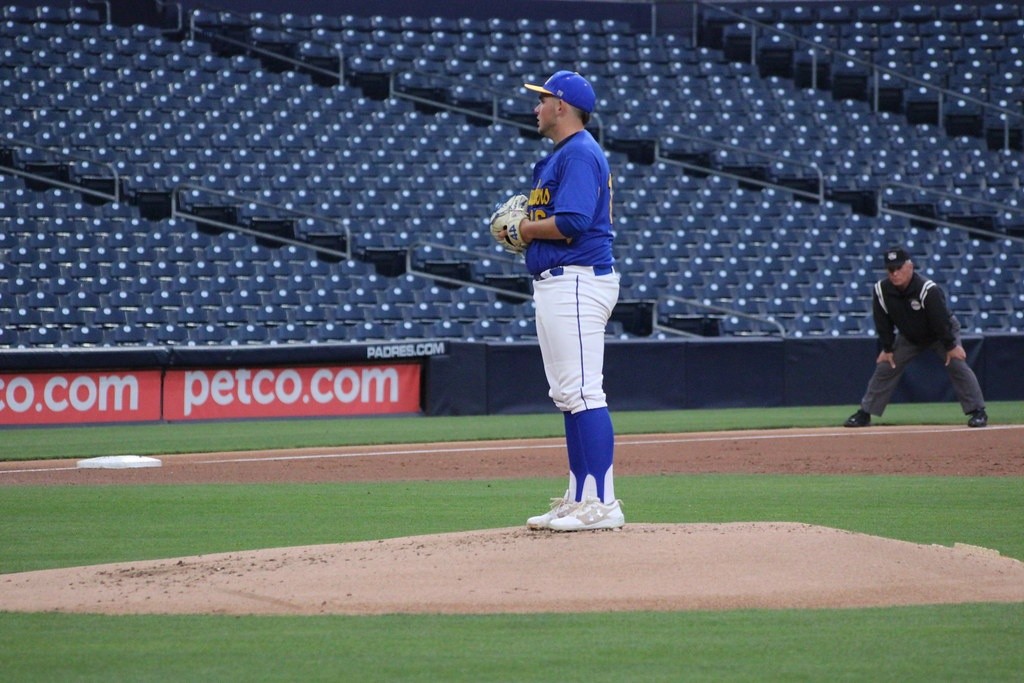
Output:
[526,491,581,530]
[968,412,988,427]
[549,497,625,532]
[844,409,871,427]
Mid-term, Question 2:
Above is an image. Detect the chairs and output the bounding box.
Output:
[1,2,1024,352]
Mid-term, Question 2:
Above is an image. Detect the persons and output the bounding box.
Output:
[488,70,626,532]
[842,248,988,427]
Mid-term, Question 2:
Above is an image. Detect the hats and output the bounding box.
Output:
[524,69,596,112]
[881,249,910,268]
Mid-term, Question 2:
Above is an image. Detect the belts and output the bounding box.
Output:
[538,263,613,281]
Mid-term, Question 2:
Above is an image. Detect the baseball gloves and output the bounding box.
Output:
[487,194,531,254]
